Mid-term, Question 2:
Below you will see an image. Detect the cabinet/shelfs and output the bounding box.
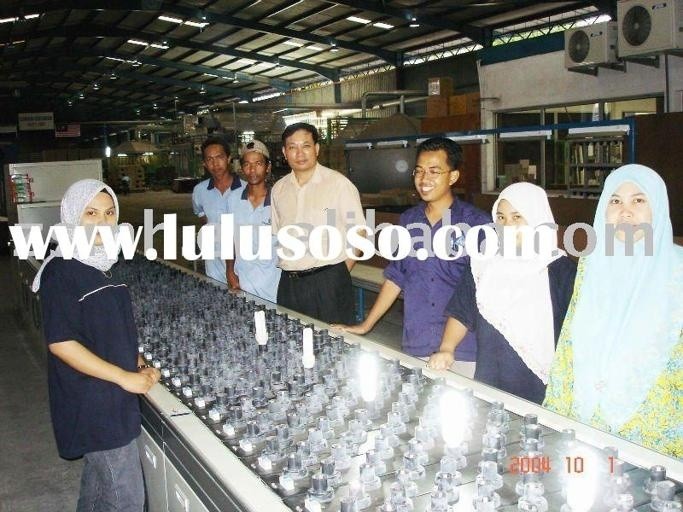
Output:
[164,442,220,512]
[564,136,623,199]
[137,414,167,512]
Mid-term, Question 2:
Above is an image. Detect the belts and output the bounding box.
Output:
[282,265,328,280]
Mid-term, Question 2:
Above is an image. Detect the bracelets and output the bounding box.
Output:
[137,363,151,369]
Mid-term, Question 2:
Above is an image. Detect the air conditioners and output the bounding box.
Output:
[564,22,624,68]
[617,0,683,58]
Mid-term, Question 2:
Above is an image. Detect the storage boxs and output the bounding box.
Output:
[425,78,480,118]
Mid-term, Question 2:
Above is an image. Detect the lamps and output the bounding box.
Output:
[345,124,630,151]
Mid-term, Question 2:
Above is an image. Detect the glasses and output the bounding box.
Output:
[413,169,449,178]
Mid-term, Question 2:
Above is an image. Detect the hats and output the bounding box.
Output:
[240,140,269,159]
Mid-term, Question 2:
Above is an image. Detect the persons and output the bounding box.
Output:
[326,135,495,382]
[118,172,132,197]
[536,162,682,464]
[268,121,370,326]
[424,181,579,407]
[188,136,249,286]
[28,178,164,510]
[221,139,282,303]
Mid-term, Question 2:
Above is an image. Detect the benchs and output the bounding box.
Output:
[350,262,404,323]
